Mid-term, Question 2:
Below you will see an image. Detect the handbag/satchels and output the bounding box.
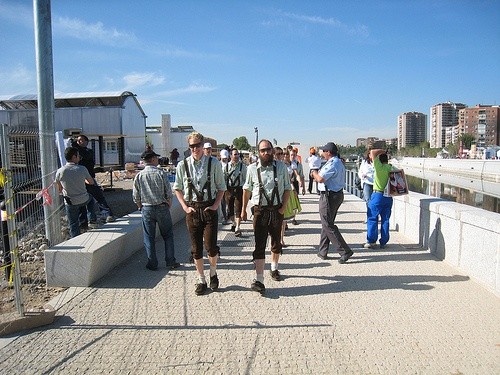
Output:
[382,170,409,197]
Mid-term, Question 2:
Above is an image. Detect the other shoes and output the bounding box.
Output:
[146,262,158,270]
[222,211,248,237]
[105,213,116,222]
[79,226,90,231]
[166,262,180,268]
[292,220,298,225]
[361,243,376,248]
[90,216,97,224]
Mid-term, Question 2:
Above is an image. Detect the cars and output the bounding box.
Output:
[440,153,449,159]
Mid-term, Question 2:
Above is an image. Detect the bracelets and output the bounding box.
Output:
[311,169,316,177]
[138,205,143,211]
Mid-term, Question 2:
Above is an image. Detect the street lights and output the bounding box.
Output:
[254,127,260,154]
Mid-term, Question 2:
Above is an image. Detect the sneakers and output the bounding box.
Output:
[251,281,265,297]
[338,251,353,264]
[270,268,281,281]
[317,252,328,260]
[210,271,218,291]
[194,283,207,294]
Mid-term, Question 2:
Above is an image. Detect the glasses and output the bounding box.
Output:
[258,147,272,152]
[188,142,202,148]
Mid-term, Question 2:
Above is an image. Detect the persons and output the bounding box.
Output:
[174,131,228,294]
[53,147,95,240]
[142,142,193,166]
[358,148,384,223]
[310,142,354,264]
[217,149,234,225]
[240,145,306,247]
[360,147,401,250]
[67,134,115,223]
[239,139,292,294]
[222,148,247,238]
[132,149,181,270]
[306,147,320,195]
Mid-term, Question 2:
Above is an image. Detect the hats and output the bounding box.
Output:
[220,149,228,158]
[203,142,212,148]
[320,142,338,153]
[141,150,161,159]
